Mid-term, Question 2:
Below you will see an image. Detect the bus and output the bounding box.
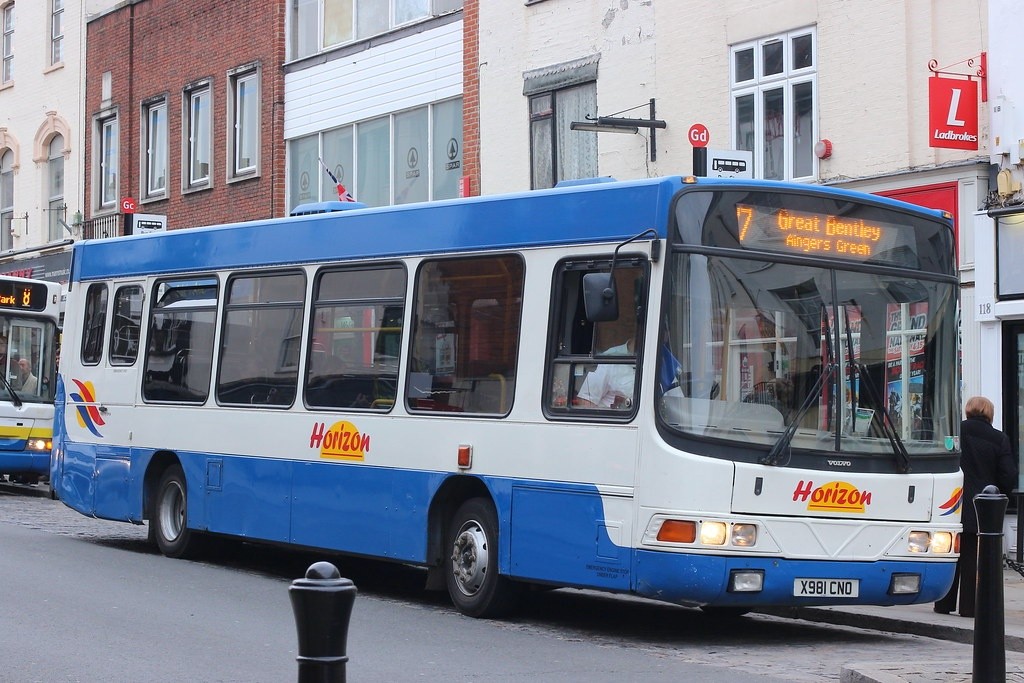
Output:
[0,275,64,484]
[47,174,967,620]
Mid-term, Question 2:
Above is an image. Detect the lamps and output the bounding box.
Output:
[815,139,832,159]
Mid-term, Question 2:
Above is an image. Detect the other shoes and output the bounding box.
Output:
[0,473,7,482]
[13,479,29,485]
[933,607,949,615]
[30,480,38,487]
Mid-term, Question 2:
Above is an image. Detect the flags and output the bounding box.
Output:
[326,168,355,203]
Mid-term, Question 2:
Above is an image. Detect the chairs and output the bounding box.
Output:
[366,358,597,416]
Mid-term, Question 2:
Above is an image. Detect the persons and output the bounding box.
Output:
[308,355,368,407]
[576,309,688,425]
[0,335,23,392]
[56,348,60,371]
[933,395,1014,618]
[19,358,37,395]
